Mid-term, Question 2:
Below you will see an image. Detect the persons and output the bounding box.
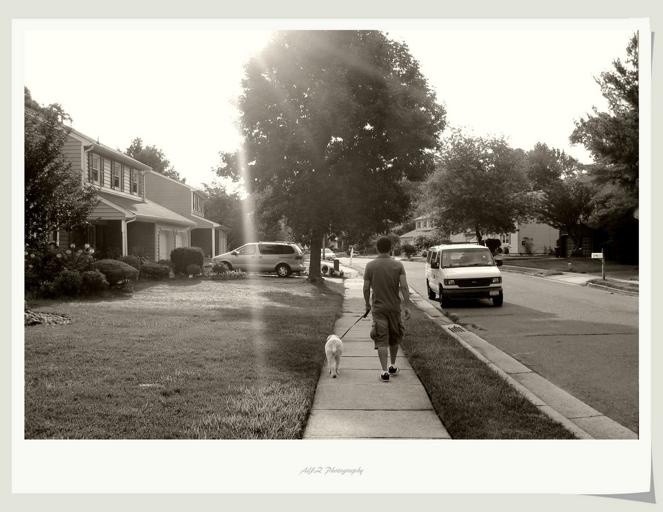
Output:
[362,236,411,382]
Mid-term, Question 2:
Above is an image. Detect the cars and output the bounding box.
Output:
[320,248,335,259]
[213,242,304,278]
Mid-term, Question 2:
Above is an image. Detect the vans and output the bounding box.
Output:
[425,244,503,308]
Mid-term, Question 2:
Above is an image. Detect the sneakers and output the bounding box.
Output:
[389,365,399,374]
[381,373,389,381]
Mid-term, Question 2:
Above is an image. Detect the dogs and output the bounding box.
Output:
[323,333,343,379]
